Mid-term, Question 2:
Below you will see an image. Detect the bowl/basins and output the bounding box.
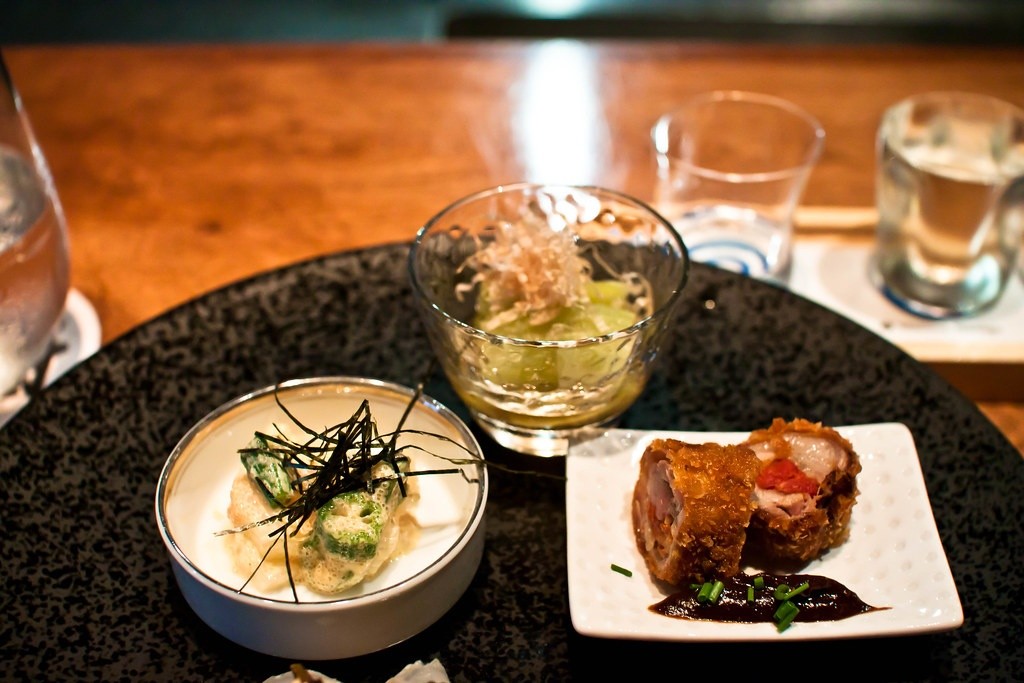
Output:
[156,376,490,662]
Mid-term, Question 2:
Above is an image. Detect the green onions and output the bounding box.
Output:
[690,578,808,632]
[611,564,632,576]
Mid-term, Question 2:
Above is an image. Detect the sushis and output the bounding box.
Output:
[634,440,755,582]
[740,422,859,562]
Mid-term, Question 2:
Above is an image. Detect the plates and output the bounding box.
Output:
[563,423,965,638]
[0,241,1024,683]
[785,208,1024,364]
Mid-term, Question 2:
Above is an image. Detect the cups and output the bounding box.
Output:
[413,183,690,455]
[871,92,1024,318]
[651,91,825,279]
[0,57,71,396]
